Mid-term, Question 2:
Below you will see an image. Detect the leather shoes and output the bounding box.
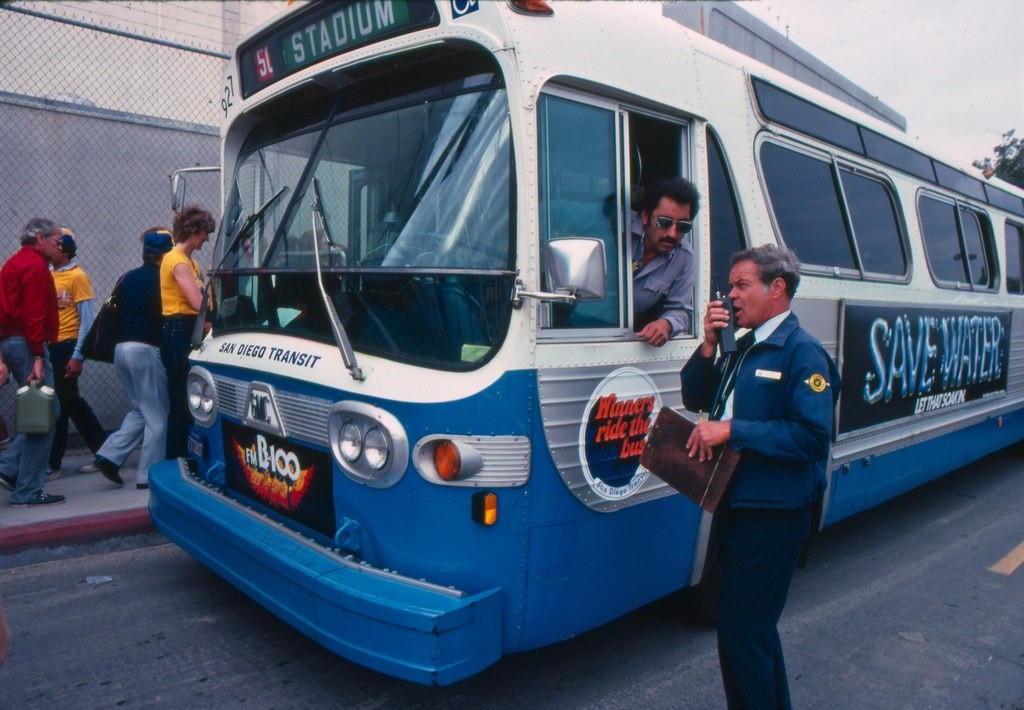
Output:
[0,473,15,490]
[11,491,66,508]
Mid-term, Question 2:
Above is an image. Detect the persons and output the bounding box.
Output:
[241,228,347,296]
[537,176,700,347]
[680,245,842,710]
[0,206,215,508]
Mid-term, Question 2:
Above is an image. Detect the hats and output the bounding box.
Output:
[143,230,174,252]
[59,227,77,258]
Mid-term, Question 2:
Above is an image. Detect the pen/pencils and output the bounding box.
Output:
[698,409,703,423]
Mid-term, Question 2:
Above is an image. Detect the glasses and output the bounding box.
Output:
[52,236,64,245]
[650,214,693,235]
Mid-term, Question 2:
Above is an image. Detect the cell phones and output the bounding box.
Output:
[713,273,738,354]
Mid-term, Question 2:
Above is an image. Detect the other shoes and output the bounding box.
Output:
[79,462,102,474]
[136,483,148,489]
[44,469,61,482]
[94,456,125,485]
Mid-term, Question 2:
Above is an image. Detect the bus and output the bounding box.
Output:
[147,0,1024,681]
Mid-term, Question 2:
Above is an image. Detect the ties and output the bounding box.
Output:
[711,331,754,421]
